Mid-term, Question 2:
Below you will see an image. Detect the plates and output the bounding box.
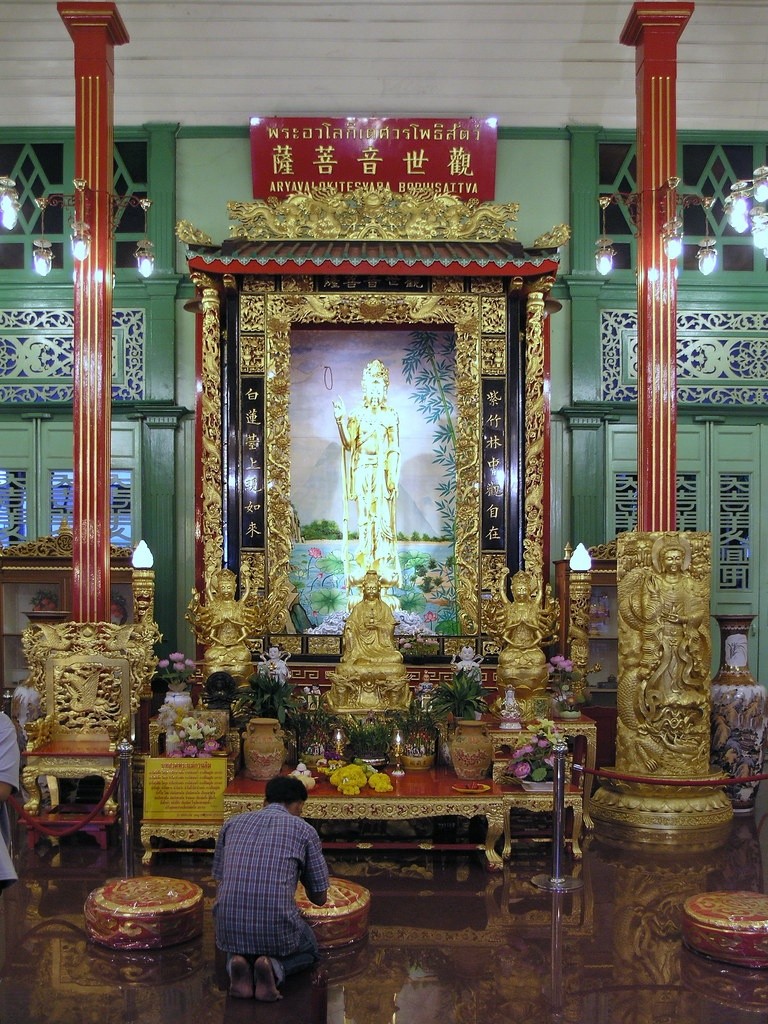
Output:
[453,783,490,793]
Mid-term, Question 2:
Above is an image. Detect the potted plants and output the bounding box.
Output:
[428,666,491,766]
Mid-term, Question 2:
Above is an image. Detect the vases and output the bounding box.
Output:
[241,717,288,781]
[520,780,554,792]
[163,689,191,716]
[450,721,493,780]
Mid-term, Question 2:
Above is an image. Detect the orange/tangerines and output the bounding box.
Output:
[353,757,374,779]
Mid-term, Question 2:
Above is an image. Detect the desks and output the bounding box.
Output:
[222,763,505,874]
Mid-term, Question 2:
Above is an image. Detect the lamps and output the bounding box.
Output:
[595,164,768,274]
[0,176,153,287]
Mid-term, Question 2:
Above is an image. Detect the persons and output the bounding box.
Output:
[416,672,437,694]
[185,569,259,667]
[340,569,403,664]
[451,645,483,685]
[310,682,323,696]
[501,684,519,712]
[331,360,401,564]
[485,568,563,669]
[211,776,331,1002]
[301,686,313,695]
[641,544,706,692]
[258,645,290,686]
[0,711,19,897]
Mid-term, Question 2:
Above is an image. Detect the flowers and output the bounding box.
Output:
[157,653,197,692]
[163,717,224,757]
[543,654,579,700]
[317,751,348,777]
[507,718,574,784]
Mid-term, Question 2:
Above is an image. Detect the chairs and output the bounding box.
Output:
[20,619,163,858]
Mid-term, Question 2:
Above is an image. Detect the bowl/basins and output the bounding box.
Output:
[20,612,72,621]
